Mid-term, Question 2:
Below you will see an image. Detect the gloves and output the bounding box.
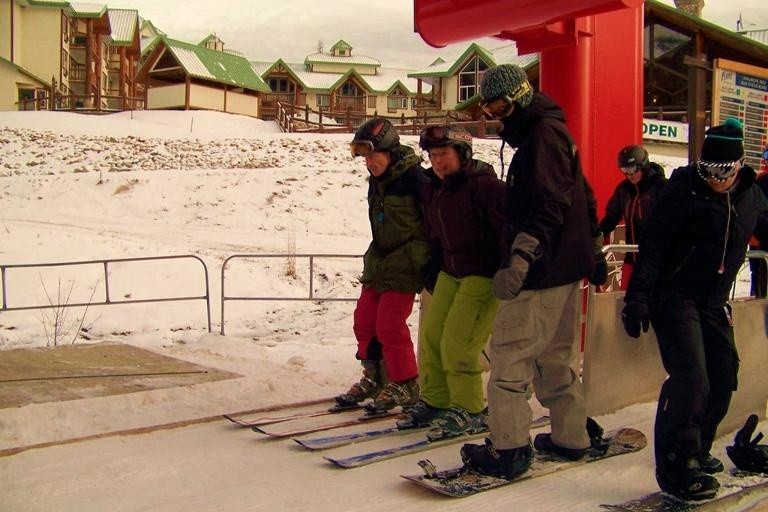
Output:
[492,232,545,300]
[587,231,607,285]
[621,301,649,338]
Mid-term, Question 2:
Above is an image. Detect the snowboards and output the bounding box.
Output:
[599,413,768,512]
[399,429,647,499]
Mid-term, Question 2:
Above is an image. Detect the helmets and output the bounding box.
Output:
[618,146,650,176]
[421,125,472,169]
[354,118,400,154]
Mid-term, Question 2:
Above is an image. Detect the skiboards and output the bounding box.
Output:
[220,401,411,437]
[222,394,551,468]
[292,418,539,469]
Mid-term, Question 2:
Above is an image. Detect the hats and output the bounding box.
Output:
[700,118,744,163]
[481,64,533,109]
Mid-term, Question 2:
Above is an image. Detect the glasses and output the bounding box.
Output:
[419,126,472,147]
[696,153,746,184]
[618,150,648,176]
[349,120,391,159]
[478,79,532,120]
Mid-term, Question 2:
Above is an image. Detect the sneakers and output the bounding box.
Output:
[348,359,386,401]
[410,399,447,423]
[700,453,724,474]
[460,437,532,479]
[657,468,720,501]
[534,433,584,461]
[376,381,420,411]
[435,406,489,437]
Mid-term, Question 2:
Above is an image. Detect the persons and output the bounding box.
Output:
[334,117,430,413]
[620,117,768,502]
[396,123,509,443]
[460,64,609,480]
[749,147,768,300]
[597,144,669,292]
